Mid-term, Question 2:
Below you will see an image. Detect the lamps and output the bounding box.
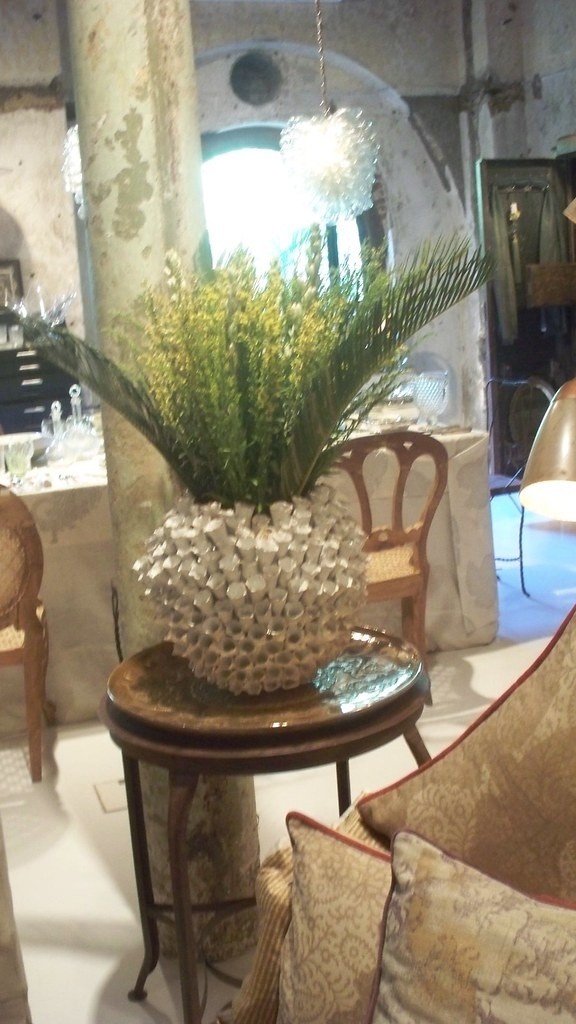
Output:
[521,376,576,522]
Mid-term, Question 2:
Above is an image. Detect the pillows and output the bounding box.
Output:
[277,811,393,1024]
[366,829,576,1024]
[357,604,576,904]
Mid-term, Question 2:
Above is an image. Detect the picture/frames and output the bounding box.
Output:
[0,259,24,323]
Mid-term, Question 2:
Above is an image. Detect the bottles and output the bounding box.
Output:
[65,383,98,460]
[45,401,76,467]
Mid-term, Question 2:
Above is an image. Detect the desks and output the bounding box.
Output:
[103,626,432,1024]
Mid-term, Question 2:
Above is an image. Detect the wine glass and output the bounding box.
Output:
[414,376,449,427]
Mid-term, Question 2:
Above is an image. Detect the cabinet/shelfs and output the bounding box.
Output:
[0,341,80,435]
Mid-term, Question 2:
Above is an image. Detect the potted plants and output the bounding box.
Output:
[0,221,510,692]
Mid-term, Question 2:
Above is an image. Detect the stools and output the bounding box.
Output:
[488,474,531,600]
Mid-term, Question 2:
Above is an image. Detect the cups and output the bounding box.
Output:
[5,437,34,477]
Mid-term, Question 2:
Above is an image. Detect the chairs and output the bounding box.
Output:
[329,431,450,707]
[0,483,51,786]
